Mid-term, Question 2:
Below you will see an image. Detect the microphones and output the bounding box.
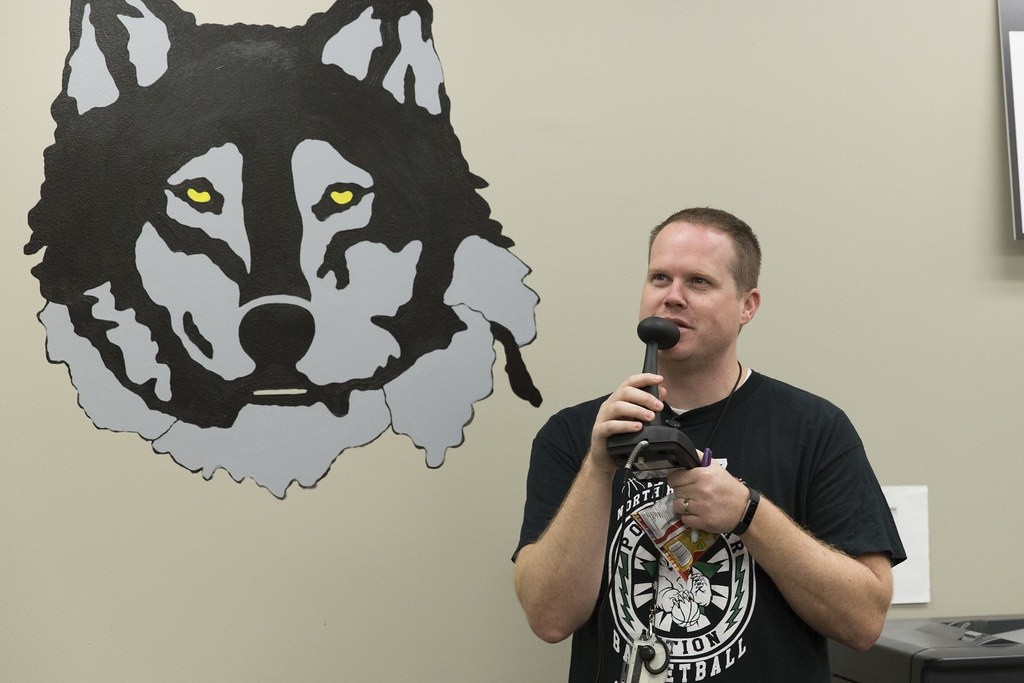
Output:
[607,316,702,481]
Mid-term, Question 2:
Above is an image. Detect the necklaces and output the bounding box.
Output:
[704,361,743,454]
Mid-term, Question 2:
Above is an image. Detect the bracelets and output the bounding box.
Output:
[729,485,760,537]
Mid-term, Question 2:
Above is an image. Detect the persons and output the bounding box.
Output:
[510,205,908,683]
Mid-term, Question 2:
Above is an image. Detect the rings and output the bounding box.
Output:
[683,498,690,512]
